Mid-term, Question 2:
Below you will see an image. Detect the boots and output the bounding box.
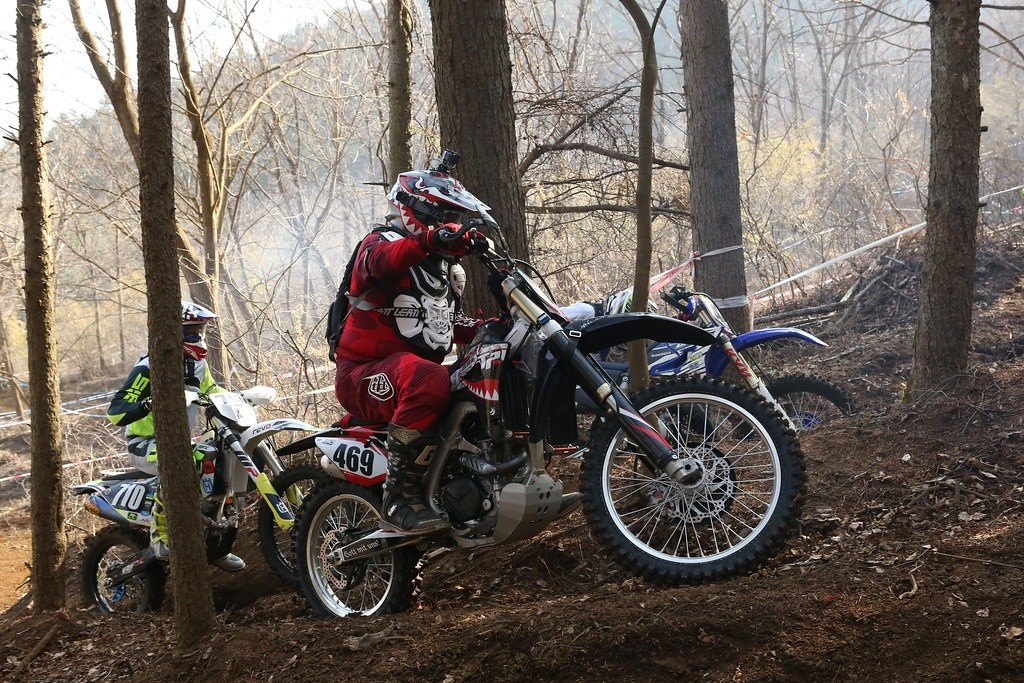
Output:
[551,492,583,521]
[208,553,246,572]
[378,422,451,535]
[148,482,169,560]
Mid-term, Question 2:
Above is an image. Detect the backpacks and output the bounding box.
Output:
[325,226,406,362]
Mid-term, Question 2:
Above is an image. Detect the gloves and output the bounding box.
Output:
[426,223,485,257]
[141,396,152,412]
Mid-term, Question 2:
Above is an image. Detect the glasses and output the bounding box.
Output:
[182,324,204,343]
[436,209,465,227]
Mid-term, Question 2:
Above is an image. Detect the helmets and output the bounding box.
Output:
[182,301,220,361]
[384,169,492,265]
[606,286,658,316]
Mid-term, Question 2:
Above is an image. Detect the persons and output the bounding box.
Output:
[108,299,280,575]
[334,165,498,536]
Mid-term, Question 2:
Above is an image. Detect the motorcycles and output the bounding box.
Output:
[71,385,366,618]
[288,207,808,622]
[531,277,860,444]
